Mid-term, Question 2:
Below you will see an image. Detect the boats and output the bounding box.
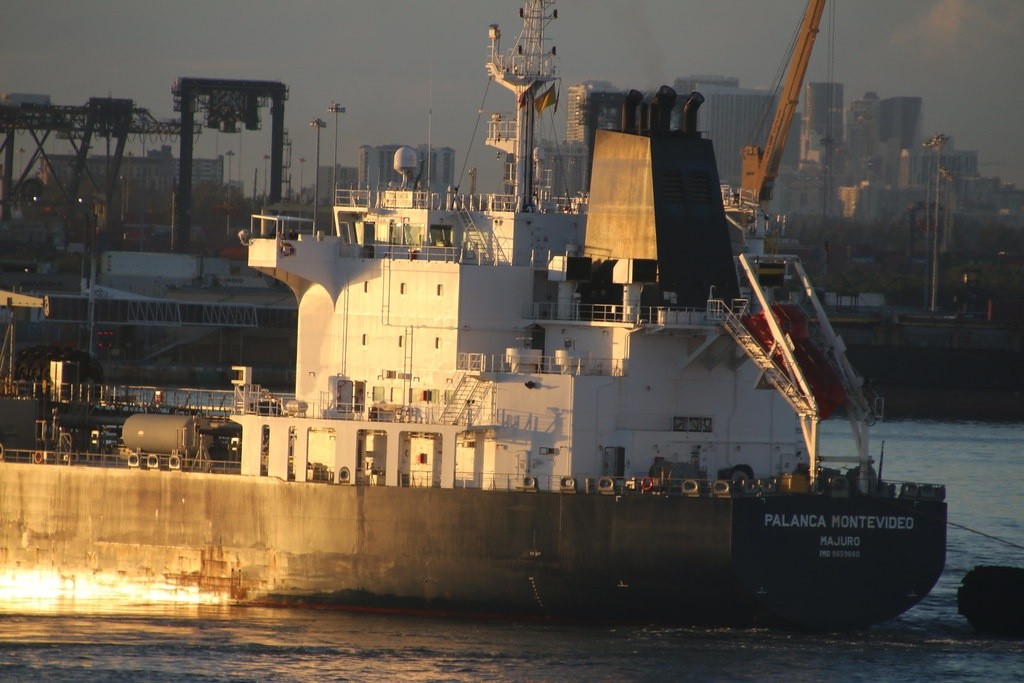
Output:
[0,0,948,633]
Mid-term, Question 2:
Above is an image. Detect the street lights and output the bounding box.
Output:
[308,119,326,238]
[327,103,345,235]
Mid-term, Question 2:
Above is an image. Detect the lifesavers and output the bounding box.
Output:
[560,476,577,489]
[597,476,614,489]
[639,476,654,492]
[832,475,849,489]
[683,479,697,494]
[901,483,947,501]
[35,450,43,463]
[522,476,537,488]
[714,481,729,494]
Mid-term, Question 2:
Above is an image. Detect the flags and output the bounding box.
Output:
[534,84,557,117]
